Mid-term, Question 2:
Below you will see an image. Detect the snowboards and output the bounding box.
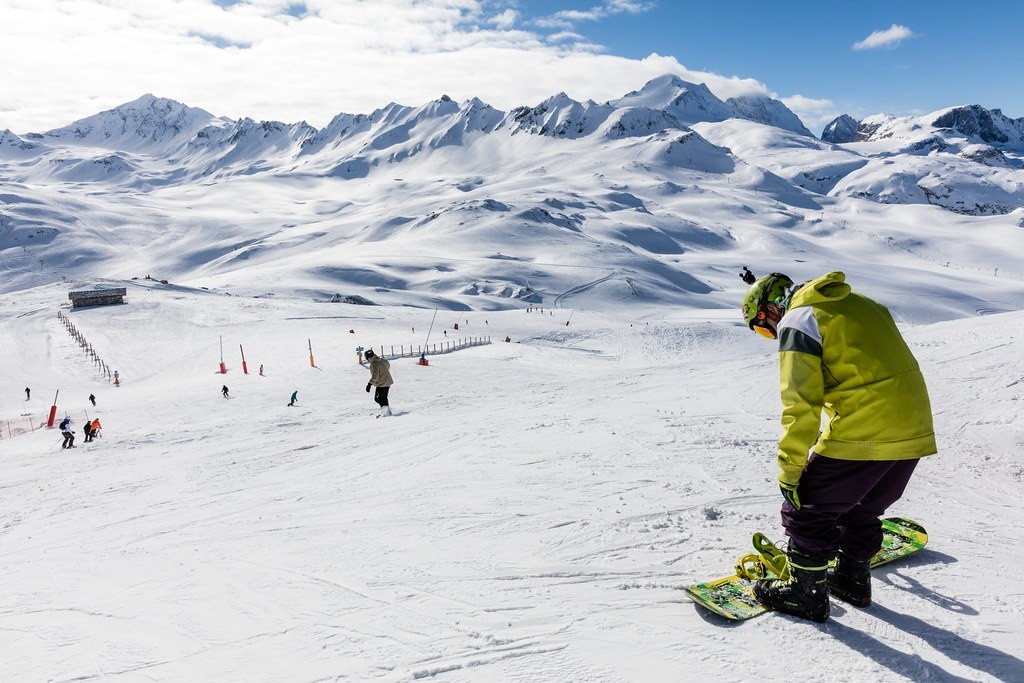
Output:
[684,517,929,620]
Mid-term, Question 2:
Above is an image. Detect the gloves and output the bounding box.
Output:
[779,480,801,511]
[366,383,372,392]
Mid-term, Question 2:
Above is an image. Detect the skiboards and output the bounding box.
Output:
[84,440,93,443]
[61,445,77,450]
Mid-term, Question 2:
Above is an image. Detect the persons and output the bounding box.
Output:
[742,269,937,624]
[61,416,76,448]
[89,394,96,406]
[287,390,298,406]
[91,418,102,437]
[25,387,31,400]
[83,421,93,442]
[221,385,229,398]
[259,364,263,375]
[364,350,394,418]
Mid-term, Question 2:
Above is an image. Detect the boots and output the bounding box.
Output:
[826,550,871,608]
[752,555,831,623]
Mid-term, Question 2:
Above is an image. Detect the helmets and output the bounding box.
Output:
[365,350,374,360]
[741,273,794,332]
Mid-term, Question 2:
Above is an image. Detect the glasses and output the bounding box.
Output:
[753,319,778,340]
